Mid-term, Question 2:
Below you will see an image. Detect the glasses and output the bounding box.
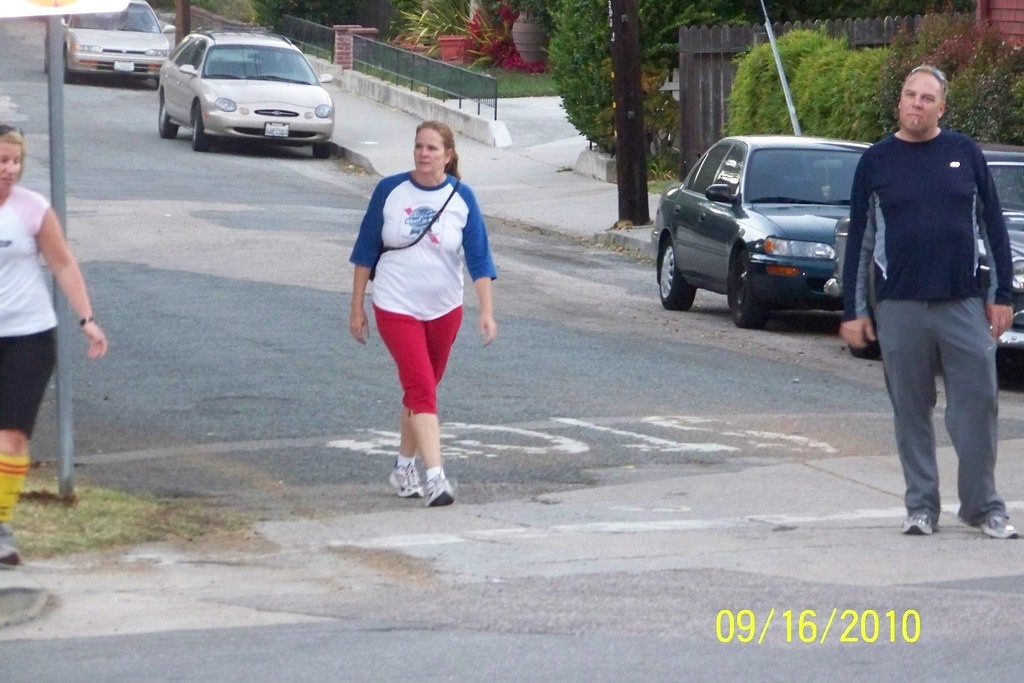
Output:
[0,125,23,136]
[910,66,946,99]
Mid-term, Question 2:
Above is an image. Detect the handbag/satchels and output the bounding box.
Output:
[368,240,387,282]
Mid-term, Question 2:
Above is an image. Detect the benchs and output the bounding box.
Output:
[208,61,261,75]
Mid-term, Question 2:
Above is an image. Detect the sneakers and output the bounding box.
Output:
[426,467,455,507]
[902,510,939,534]
[957,514,1018,538]
[389,458,423,498]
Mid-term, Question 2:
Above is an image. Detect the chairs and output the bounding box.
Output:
[69,13,102,29]
[751,172,787,199]
[829,167,856,200]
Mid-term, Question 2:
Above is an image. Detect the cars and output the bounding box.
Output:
[44,0,176,87]
[833,138,1024,374]
[651,138,877,332]
[157,28,336,161]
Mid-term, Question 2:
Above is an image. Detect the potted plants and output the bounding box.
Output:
[512,0,558,64]
[400,0,475,62]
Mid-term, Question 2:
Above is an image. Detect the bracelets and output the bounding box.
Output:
[79,317,95,326]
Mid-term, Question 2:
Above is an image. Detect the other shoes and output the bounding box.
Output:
[0,522,21,565]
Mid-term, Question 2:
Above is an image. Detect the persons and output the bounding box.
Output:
[840,64,1019,539]
[0,122,107,565]
[350,120,498,508]
